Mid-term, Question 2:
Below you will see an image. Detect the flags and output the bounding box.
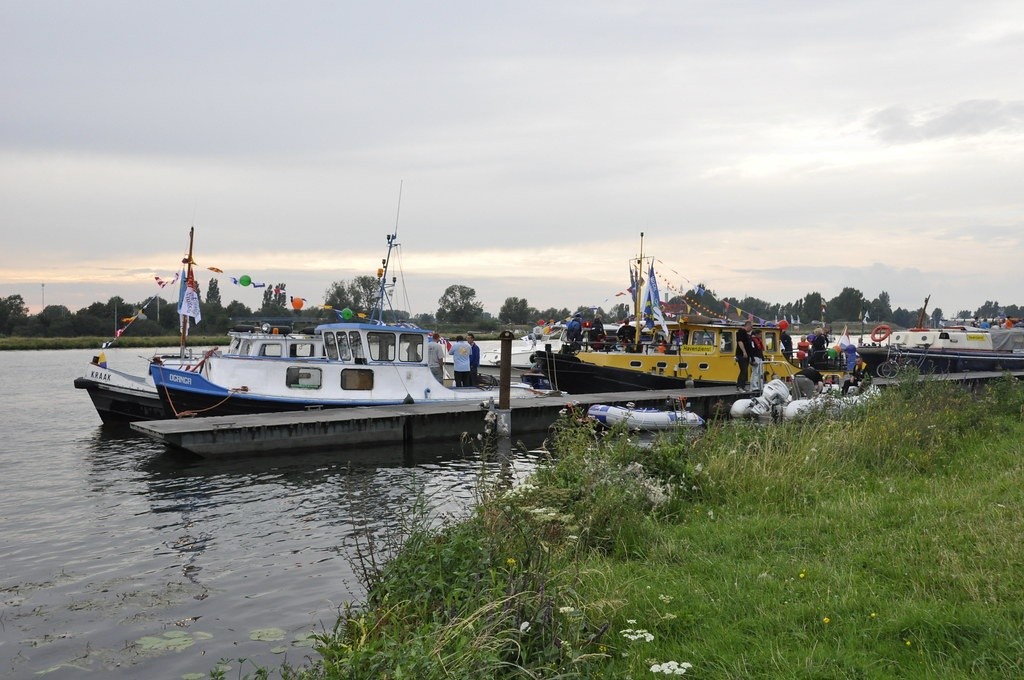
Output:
[176,266,201,336]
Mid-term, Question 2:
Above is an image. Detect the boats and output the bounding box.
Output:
[148,180,563,422]
[69,226,327,424]
[730,381,881,427]
[584,404,705,432]
[520,231,870,395]
[891,325,1024,356]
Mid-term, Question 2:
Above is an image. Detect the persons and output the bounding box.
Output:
[617,318,636,354]
[428,333,444,387]
[465,332,480,387]
[588,314,606,352]
[449,335,472,387]
[972,315,1024,329]
[567,314,583,353]
[735,321,872,398]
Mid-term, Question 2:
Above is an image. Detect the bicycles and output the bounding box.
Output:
[876,353,917,379]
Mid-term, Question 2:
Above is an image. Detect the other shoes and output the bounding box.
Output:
[735,387,747,393]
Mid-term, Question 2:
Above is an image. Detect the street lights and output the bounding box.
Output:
[41,283,45,311]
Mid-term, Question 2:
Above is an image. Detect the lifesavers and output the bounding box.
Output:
[871,325,891,342]
[530,354,538,363]
